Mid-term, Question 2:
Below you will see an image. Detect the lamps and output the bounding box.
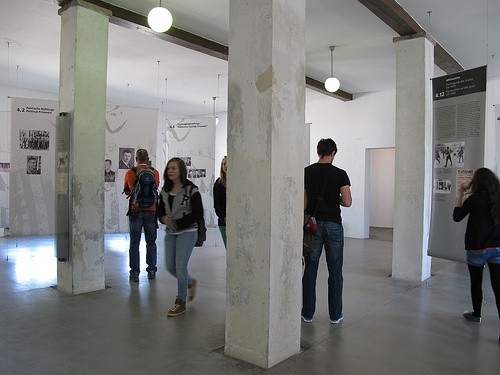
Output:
[324,46,340,92]
[147,0,173,33]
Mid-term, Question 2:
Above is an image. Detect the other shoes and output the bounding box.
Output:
[146,268,157,278]
[301,308,313,322]
[130,271,139,282]
[330,315,344,323]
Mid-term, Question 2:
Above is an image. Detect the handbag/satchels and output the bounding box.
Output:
[189,184,207,246]
[303,210,318,255]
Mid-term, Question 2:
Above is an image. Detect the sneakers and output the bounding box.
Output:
[187,279,196,301]
[463,311,481,324]
[167,298,187,316]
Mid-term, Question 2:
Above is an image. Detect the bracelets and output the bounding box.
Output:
[454,205,461,208]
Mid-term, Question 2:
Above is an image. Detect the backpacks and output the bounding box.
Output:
[126,167,158,216]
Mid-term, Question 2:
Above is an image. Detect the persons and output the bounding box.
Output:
[104,159,115,181]
[19,129,50,151]
[27,158,40,174]
[214,157,226,249]
[119,148,134,169]
[185,157,206,179]
[452,168,500,324]
[301,138,352,323]
[434,144,463,168]
[157,158,207,317]
[122,148,160,282]
